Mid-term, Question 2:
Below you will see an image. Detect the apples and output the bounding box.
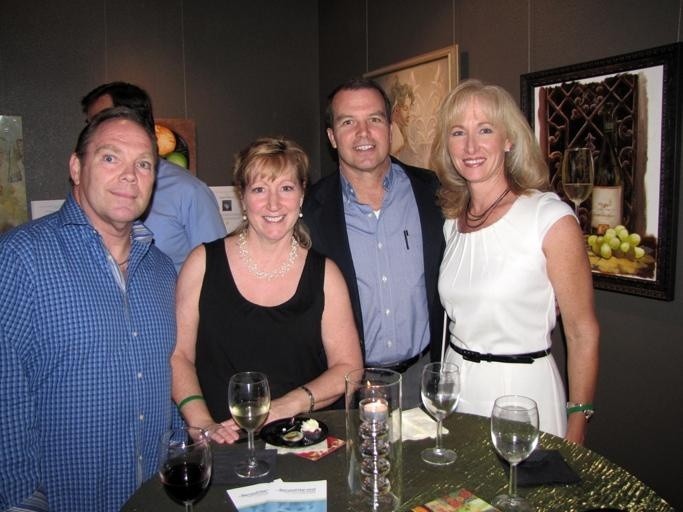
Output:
[155,124,176,158]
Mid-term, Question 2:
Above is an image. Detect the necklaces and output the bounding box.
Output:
[464,184,513,228]
[237,228,299,281]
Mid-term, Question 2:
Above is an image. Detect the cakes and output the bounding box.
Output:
[301,418,322,440]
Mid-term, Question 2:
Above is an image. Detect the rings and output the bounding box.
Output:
[198,429,209,438]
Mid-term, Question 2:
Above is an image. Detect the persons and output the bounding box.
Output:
[296,84,445,411]
[69,80,231,274]
[171,139,363,445]
[428,76,600,450]
[0,106,185,510]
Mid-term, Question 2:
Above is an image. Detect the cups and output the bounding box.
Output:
[155,424,213,511]
[344,368,403,511]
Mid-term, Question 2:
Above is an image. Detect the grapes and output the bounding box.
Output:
[587,225,646,259]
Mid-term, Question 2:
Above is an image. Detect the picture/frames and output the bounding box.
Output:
[363,43,459,168]
[520,42,683,302]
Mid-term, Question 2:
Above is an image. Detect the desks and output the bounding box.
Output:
[121,409,672,512]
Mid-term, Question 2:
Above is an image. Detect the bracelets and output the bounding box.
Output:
[564,399,593,418]
[299,385,314,412]
[177,394,208,412]
[566,401,595,420]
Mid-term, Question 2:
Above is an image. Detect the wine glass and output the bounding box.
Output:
[489,395,538,512]
[418,362,461,467]
[561,147,593,221]
[226,369,272,479]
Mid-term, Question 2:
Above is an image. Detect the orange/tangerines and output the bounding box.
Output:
[166,153,186,170]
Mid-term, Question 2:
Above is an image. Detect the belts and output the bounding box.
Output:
[449,341,551,363]
[365,344,430,375]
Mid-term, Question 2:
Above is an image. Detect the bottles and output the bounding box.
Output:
[589,104,625,237]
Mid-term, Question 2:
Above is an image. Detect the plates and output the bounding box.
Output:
[495,446,581,486]
[258,417,328,450]
